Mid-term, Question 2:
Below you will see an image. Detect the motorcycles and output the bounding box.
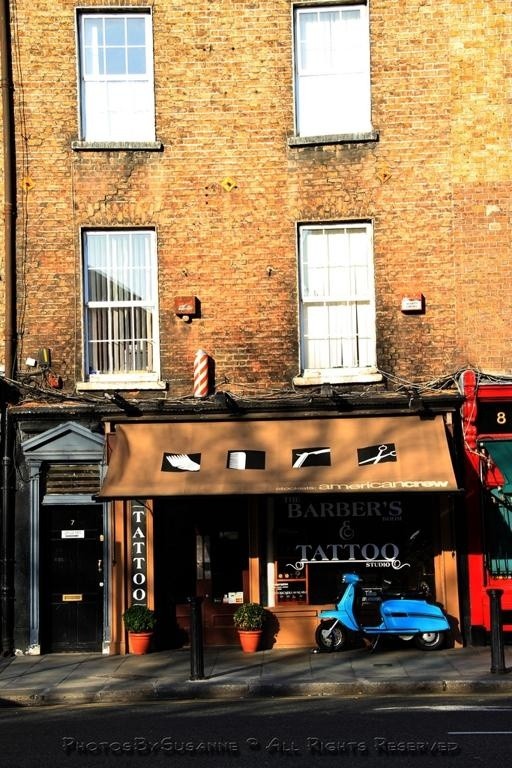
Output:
[315,570,450,652]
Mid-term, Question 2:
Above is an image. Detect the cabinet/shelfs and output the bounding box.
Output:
[275,562,309,605]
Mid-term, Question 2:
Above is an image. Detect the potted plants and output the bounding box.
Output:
[233,603,268,656]
[121,603,159,657]
